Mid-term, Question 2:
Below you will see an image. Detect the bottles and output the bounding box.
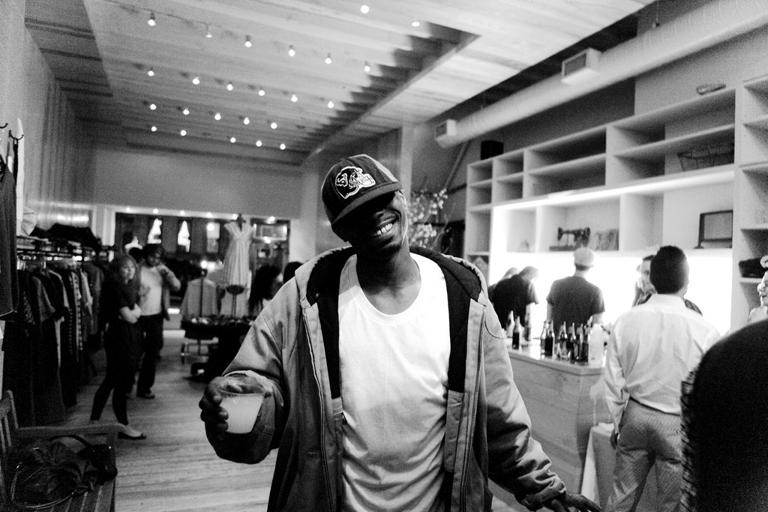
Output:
[512,315,521,350]
[540,320,555,357]
[558,321,590,364]
[506,310,515,338]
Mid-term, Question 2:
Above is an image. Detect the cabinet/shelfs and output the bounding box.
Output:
[462,74,767,343]
[488,348,608,512]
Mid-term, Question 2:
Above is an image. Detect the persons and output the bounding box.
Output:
[632,254,704,315]
[678,319,768,512]
[546,247,605,331]
[246,265,281,317]
[199,155,602,512]
[487,266,518,296]
[597,245,718,512]
[88,254,147,440]
[492,265,539,333]
[126,245,182,399]
[219,213,255,319]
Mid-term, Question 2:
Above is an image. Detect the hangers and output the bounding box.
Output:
[22,249,101,274]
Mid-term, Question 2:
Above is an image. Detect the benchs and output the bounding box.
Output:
[0,389,127,512]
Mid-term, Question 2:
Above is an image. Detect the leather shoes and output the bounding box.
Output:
[117,429,146,440]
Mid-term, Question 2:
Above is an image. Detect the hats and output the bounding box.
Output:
[574,247,595,266]
[320,152,402,224]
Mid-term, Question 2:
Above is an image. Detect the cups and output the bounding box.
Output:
[217,383,266,434]
